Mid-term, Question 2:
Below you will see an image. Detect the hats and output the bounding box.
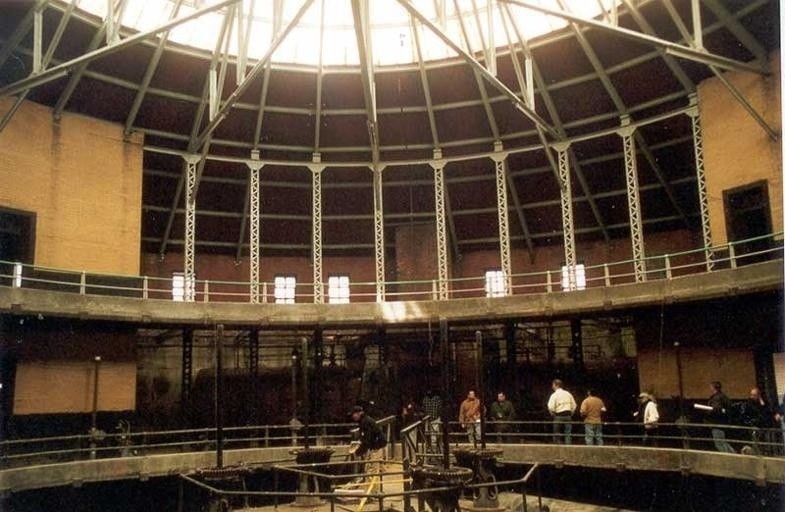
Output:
[345,404,365,417]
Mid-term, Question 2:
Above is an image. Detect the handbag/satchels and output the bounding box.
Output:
[368,432,387,451]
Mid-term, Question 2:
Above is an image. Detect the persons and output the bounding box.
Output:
[394,403,415,444]
[421,385,444,443]
[772,392,785,456]
[579,387,608,446]
[491,391,516,444]
[546,378,578,446]
[704,380,738,453]
[350,405,388,488]
[740,386,774,457]
[632,391,660,447]
[458,389,487,443]
